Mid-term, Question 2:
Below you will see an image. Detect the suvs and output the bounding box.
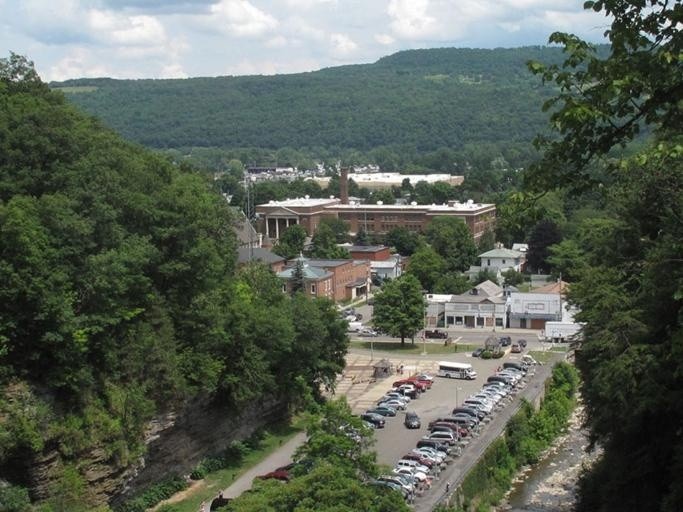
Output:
[404,411,421,429]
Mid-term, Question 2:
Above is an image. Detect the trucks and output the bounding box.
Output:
[544,321,589,344]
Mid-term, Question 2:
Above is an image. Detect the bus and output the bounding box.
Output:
[437,361,477,380]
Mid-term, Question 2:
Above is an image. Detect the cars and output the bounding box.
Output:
[253,336,538,504]
[336,308,383,338]
[421,329,450,339]
